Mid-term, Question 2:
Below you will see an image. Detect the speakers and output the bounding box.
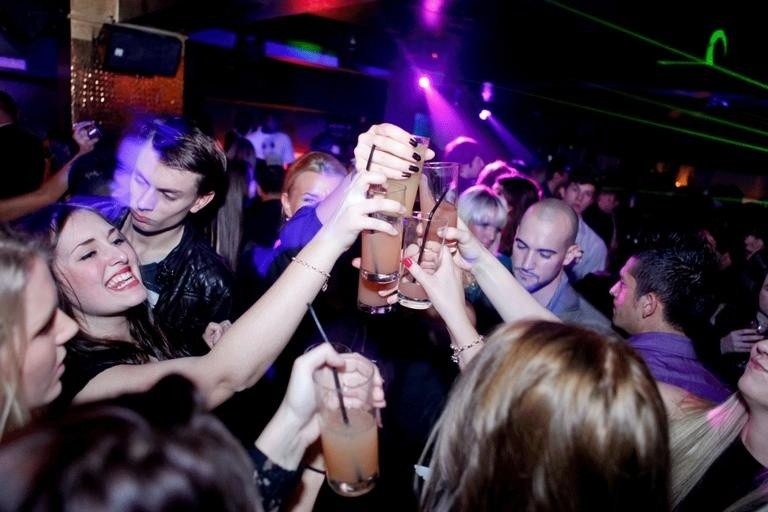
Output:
[90,23,183,79]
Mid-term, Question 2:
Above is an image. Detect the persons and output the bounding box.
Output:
[0,120,768,511]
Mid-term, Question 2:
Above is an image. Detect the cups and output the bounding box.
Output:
[396,210,448,309]
[384,136,430,216]
[360,184,404,285]
[304,342,353,353]
[312,356,380,498]
[421,160,458,254]
[356,275,394,315]
